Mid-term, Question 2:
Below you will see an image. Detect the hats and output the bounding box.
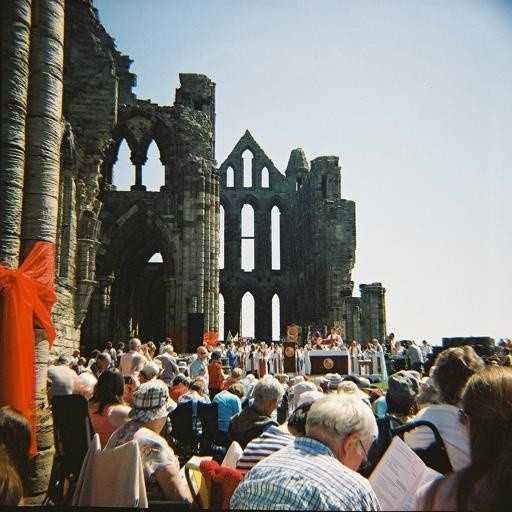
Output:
[324,372,371,386]
[387,370,420,399]
[128,380,178,422]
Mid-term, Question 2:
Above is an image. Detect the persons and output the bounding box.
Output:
[1,323,511,510]
